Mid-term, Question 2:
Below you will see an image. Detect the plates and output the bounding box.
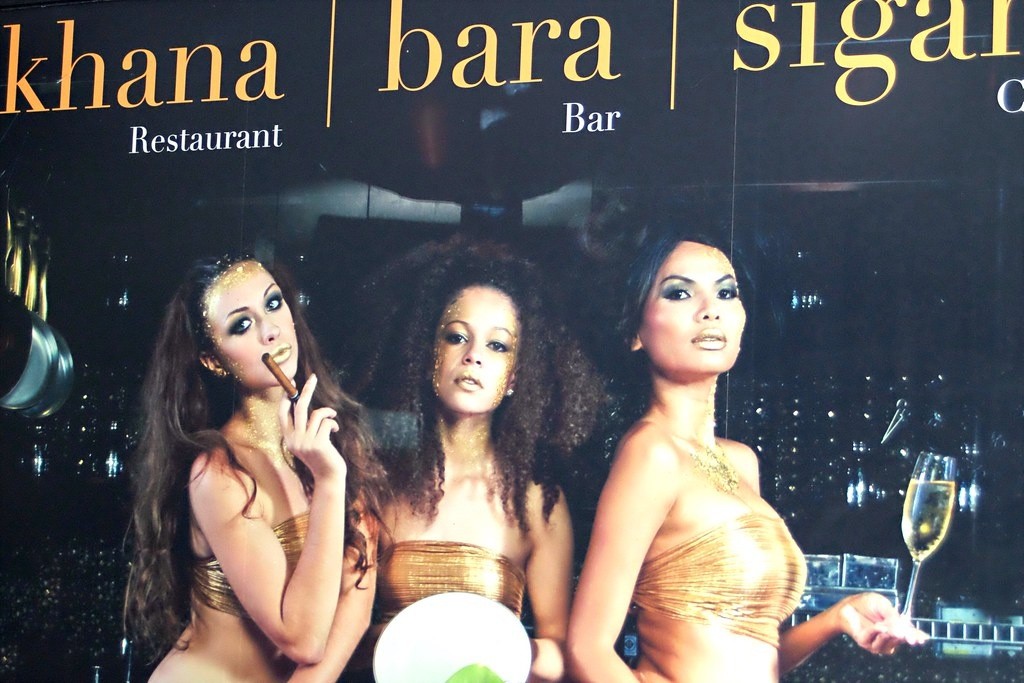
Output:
[373,591,532,683]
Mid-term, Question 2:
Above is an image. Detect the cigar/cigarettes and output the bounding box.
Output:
[261,352,314,420]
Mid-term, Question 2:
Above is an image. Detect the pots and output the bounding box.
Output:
[0,184,74,419]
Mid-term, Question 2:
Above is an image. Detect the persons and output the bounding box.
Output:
[286,241,601,683]
[123,245,396,683]
[564,233,925,683]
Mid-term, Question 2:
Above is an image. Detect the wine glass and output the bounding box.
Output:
[878,450,955,638]
